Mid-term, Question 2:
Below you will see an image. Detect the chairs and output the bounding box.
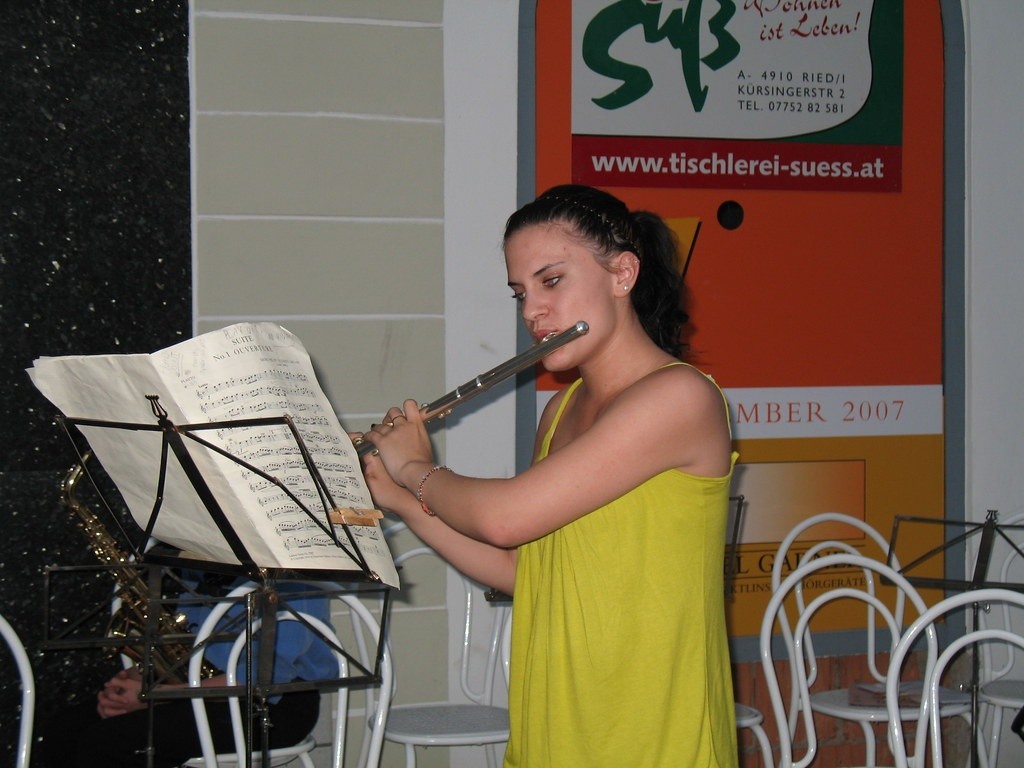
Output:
[111,520,514,768]
[732,511,1023,768]
[0,614,35,768]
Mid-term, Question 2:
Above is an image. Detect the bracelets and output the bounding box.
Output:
[417,465,454,516]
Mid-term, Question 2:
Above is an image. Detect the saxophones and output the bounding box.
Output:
[58,447,222,687]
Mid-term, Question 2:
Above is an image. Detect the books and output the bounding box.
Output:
[27,320,398,591]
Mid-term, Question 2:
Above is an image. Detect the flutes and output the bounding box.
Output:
[353,320,590,457]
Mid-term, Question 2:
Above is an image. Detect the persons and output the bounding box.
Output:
[346,180,739,767]
[43,563,339,768]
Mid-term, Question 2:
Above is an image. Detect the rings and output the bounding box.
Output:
[392,415,403,422]
